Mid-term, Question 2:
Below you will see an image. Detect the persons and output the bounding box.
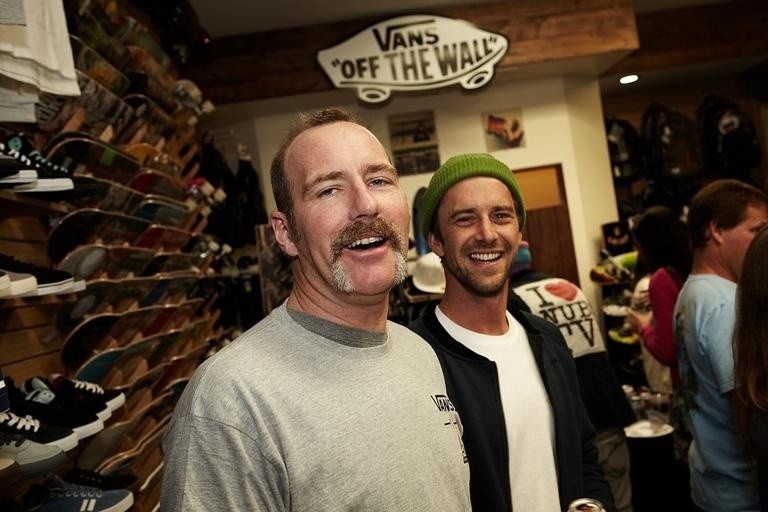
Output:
[158,106,476,511]
[407,152,618,512]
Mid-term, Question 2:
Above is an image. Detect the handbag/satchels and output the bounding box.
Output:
[601,223,632,255]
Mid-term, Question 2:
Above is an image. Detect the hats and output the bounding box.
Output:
[425,152,527,235]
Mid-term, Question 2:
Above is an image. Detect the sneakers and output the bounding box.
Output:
[1,377,125,464]
[2,256,89,303]
[0,132,73,197]
[3,475,134,512]
